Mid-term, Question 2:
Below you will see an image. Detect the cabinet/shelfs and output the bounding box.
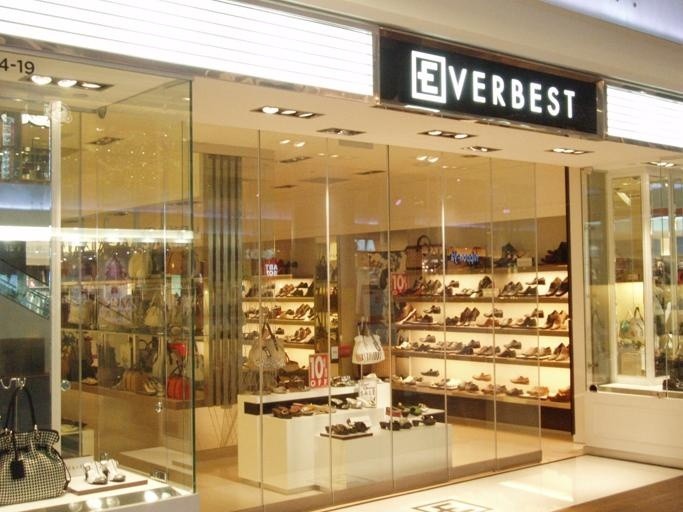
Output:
[59,265,570,498]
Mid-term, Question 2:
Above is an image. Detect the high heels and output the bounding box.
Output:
[101,459,125,481]
[326,418,369,435]
[83,461,107,484]
[263,373,304,393]
[379,402,435,430]
[276,260,298,274]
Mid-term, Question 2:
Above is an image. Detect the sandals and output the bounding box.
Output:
[272,397,377,419]
[330,375,354,387]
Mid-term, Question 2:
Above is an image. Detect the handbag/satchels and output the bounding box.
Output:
[249,322,287,371]
[404,235,439,269]
[60,238,200,281]
[351,320,385,365]
[0,387,71,506]
[60,285,203,334]
[620,307,644,339]
[62,334,199,400]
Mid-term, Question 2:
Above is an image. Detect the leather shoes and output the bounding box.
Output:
[402,304,570,329]
[541,242,568,264]
[246,282,308,297]
[396,369,571,401]
[244,303,313,320]
[401,276,568,296]
[291,328,311,341]
[493,244,519,266]
[395,335,570,360]
[663,379,682,391]
